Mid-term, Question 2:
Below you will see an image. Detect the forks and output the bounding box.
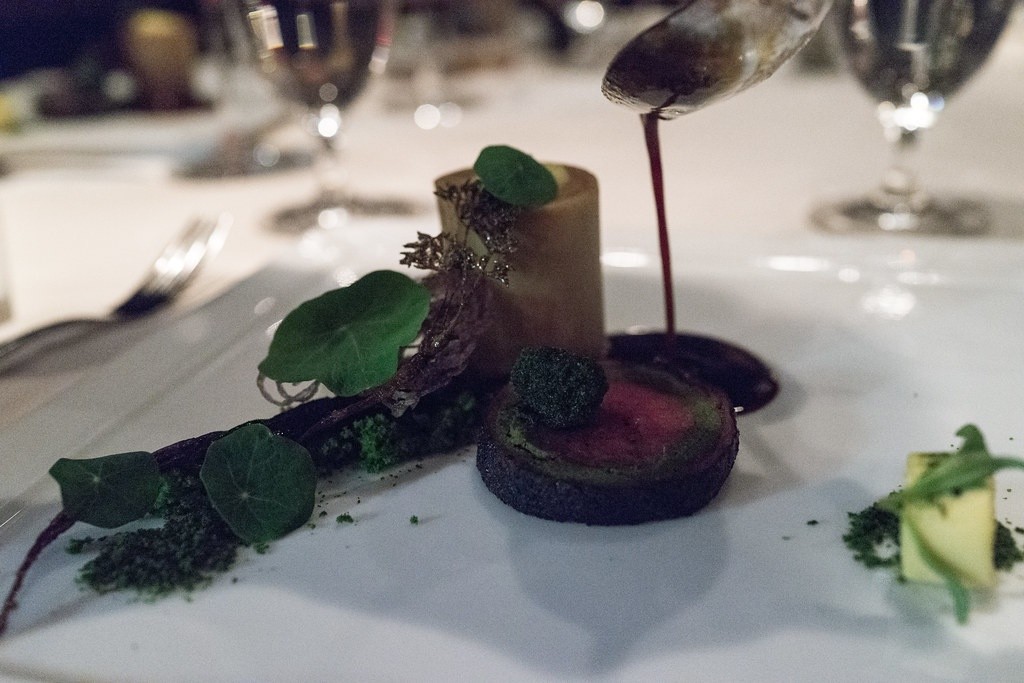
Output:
[0,217,221,370]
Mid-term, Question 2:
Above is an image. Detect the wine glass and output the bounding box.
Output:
[241,0,419,237]
[811,0,1015,235]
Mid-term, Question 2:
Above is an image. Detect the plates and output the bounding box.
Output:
[0,239,1023,682]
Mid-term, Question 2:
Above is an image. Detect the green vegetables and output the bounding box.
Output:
[880,424,1024,628]
[50,144,561,544]
